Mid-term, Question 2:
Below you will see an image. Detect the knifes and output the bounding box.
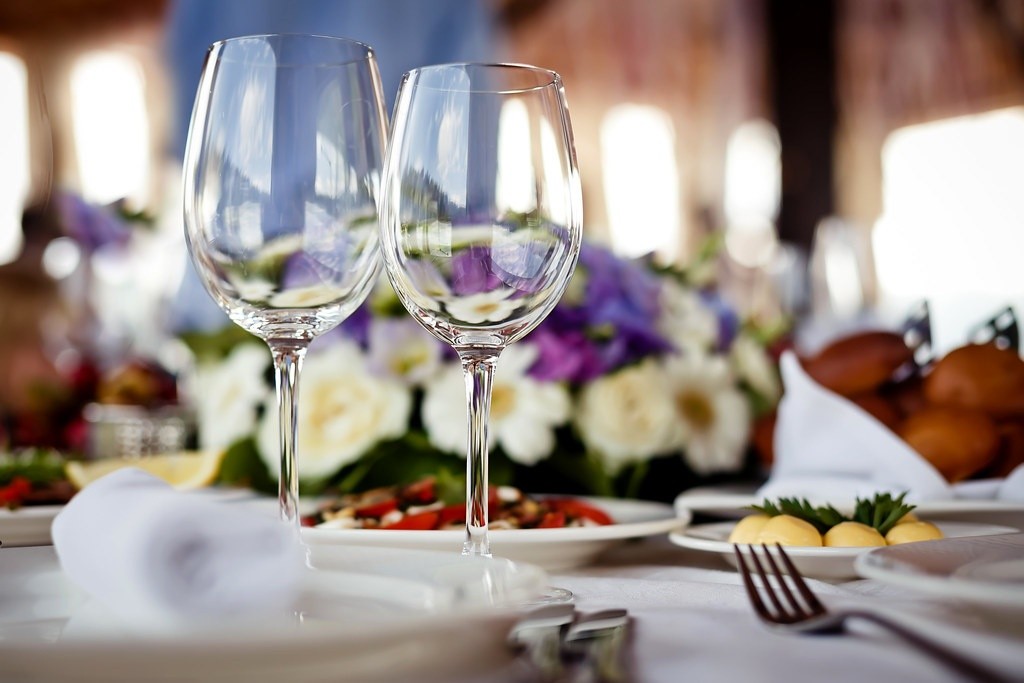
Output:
[503,604,634,681]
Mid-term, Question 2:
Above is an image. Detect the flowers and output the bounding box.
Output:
[206,185,790,507]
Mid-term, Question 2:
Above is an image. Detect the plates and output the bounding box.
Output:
[673,487,1024,515]
[300,494,692,571]
[0,504,67,548]
[667,519,1022,580]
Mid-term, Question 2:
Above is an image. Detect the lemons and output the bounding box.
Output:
[64,446,224,490]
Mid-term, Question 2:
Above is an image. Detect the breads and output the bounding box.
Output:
[802,329,1024,482]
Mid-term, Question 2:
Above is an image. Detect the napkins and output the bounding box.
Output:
[756,347,953,504]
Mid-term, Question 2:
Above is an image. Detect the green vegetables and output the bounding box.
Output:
[0,446,68,491]
[739,489,919,537]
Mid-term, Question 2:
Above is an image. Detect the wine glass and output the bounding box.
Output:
[181,32,389,532]
[376,60,584,611]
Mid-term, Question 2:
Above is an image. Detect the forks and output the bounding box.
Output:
[727,541,1019,683]
